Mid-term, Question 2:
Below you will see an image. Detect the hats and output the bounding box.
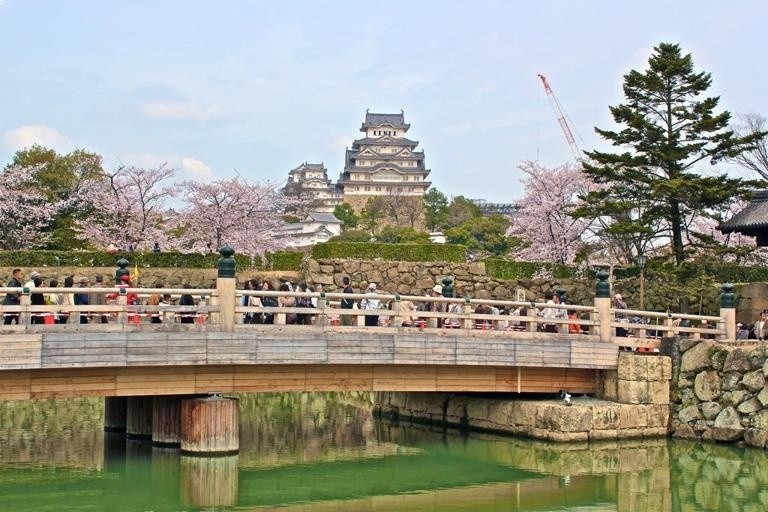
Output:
[29,272,40,277]
[432,284,443,294]
[79,276,90,284]
[367,283,377,289]
[121,275,128,282]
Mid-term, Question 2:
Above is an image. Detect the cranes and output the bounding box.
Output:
[536,73,598,195]
